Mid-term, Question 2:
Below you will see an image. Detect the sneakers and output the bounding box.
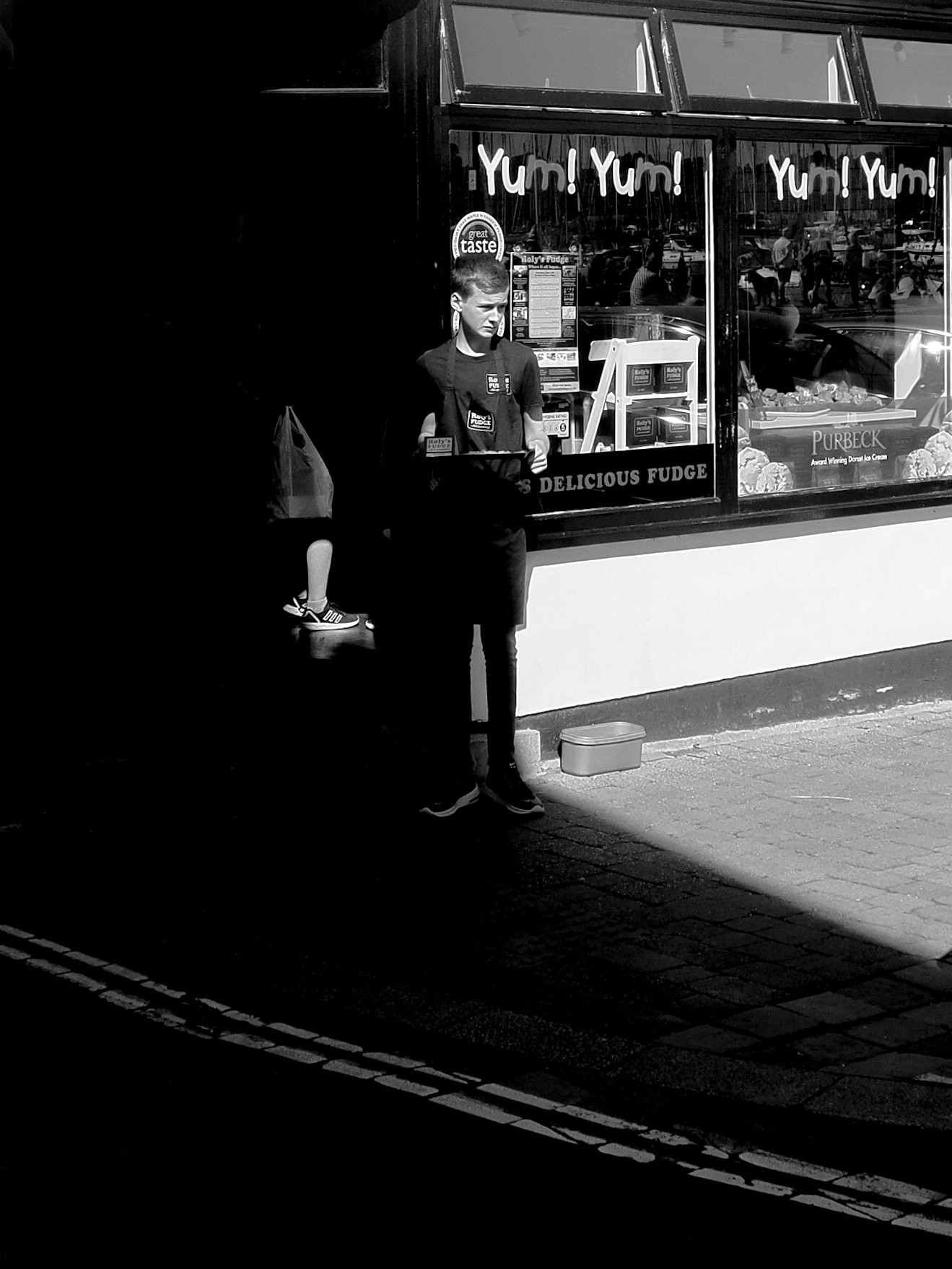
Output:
[301,599,358,632]
[420,776,479,818]
[283,592,339,616]
[481,759,544,814]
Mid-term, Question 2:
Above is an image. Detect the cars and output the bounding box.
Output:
[816,315,950,392]
[578,306,894,430]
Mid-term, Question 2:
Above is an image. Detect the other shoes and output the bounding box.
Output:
[852,302,864,308]
[827,303,839,309]
[780,298,788,304]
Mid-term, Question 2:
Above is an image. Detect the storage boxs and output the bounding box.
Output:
[558,722,650,776]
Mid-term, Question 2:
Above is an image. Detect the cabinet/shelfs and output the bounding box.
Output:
[583,337,700,453]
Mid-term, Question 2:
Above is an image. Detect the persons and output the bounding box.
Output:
[399,251,550,818]
[263,400,370,628]
[583,235,707,310]
[771,227,864,308]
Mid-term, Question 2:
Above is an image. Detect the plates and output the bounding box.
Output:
[763,407,831,417]
[456,449,527,458]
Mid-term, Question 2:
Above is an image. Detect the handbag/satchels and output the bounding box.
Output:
[266,406,335,517]
